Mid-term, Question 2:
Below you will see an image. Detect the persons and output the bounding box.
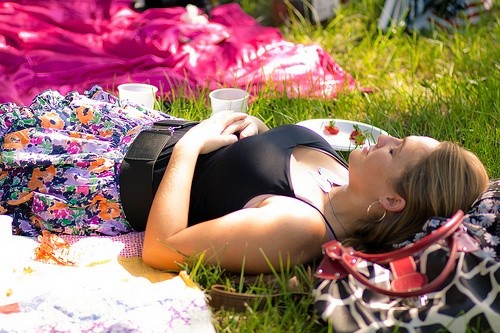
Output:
[0,85,488,274]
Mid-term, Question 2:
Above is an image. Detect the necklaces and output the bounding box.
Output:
[315,164,353,234]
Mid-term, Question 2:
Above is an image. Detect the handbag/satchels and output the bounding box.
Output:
[313,209,500,333]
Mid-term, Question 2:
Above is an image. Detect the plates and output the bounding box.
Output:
[296,118,393,152]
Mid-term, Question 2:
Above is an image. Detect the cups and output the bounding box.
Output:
[117,83,158,110]
[209,88,249,113]
